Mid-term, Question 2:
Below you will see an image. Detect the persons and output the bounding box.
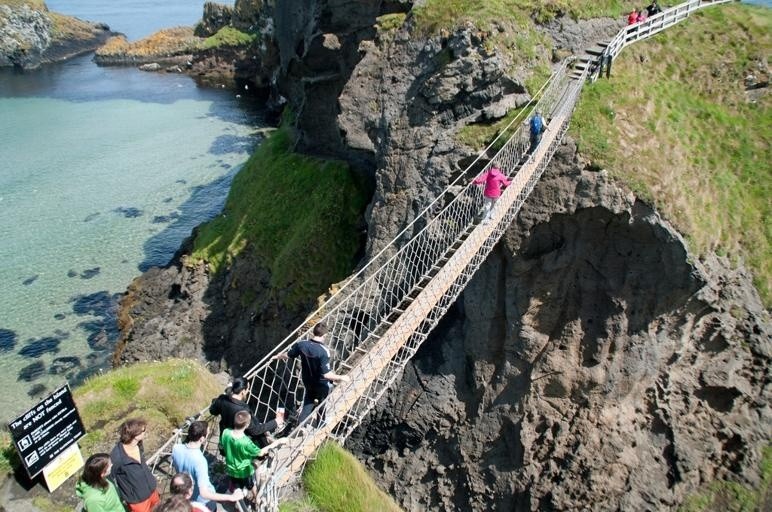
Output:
[636,8,645,23]
[74,375,286,512]
[628,8,637,24]
[270,319,354,428]
[518,107,553,152]
[472,159,512,218]
[646,0,660,17]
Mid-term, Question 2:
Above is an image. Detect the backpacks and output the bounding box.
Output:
[530,115,543,135]
